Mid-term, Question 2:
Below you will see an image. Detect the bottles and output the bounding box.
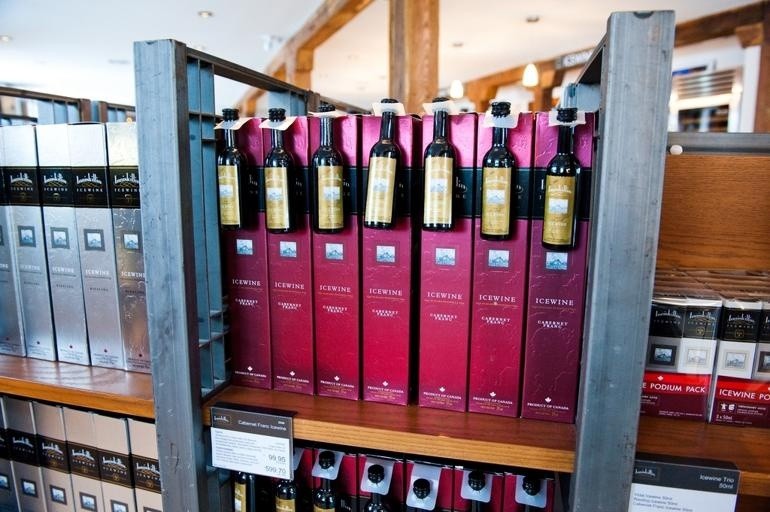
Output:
[540,106,584,254]
[362,110,403,231]
[261,107,300,236]
[421,109,458,233]
[362,463,389,512]
[232,471,258,512]
[479,100,519,242]
[275,479,298,512]
[467,470,486,512]
[308,103,347,235]
[521,475,547,512]
[312,450,338,512]
[406,478,440,512]
[215,107,274,235]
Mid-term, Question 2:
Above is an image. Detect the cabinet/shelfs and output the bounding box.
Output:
[2,38,182,511]
[150,12,636,511]
[606,4,770,512]
